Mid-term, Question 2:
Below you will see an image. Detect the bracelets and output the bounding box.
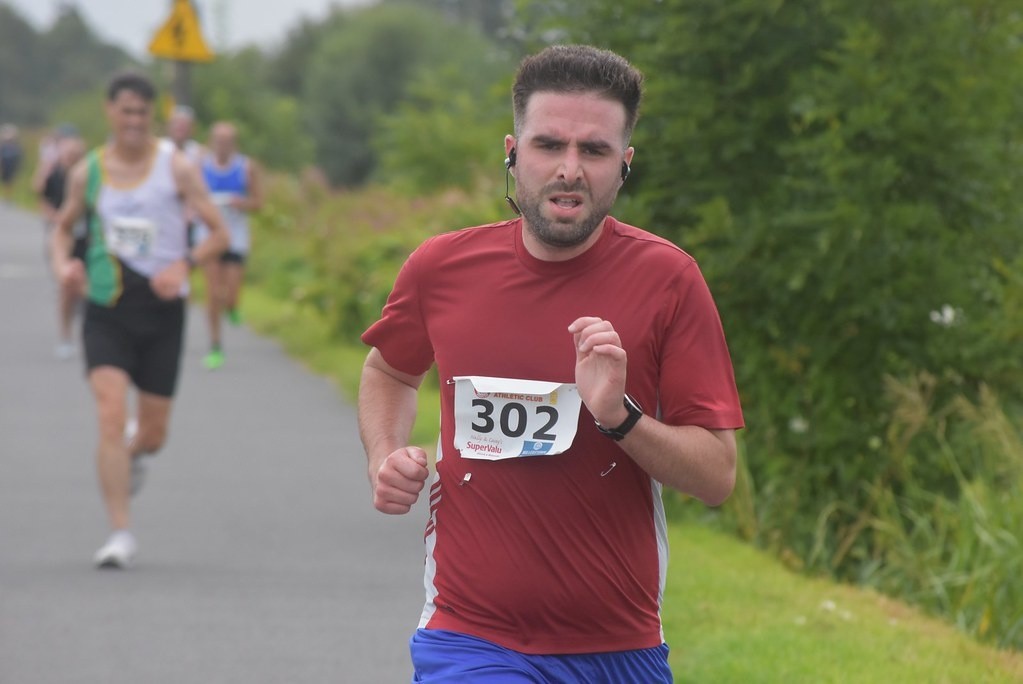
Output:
[182,253,196,269]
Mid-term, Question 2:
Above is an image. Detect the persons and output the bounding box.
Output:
[167,105,201,163]
[194,121,262,369]
[36,128,90,347]
[358,42,745,684]
[51,76,232,569]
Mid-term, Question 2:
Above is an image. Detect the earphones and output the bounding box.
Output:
[621,161,630,181]
[507,148,517,168]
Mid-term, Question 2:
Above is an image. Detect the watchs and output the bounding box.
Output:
[594,391,644,440]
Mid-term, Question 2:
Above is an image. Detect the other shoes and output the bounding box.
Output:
[205,347,223,369]
[226,307,239,323]
[125,420,148,496]
[99,531,135,569]
